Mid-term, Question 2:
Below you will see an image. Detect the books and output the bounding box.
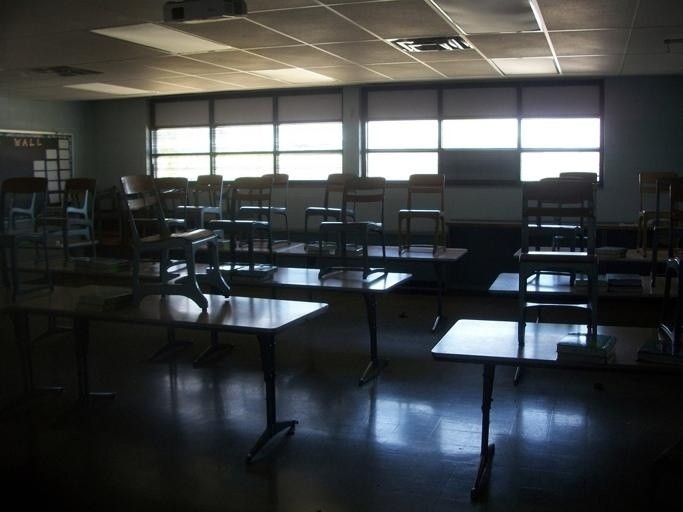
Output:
[596,247,683,365]
[70,239,349,305]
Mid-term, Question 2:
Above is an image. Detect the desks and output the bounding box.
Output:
[427,316,681,502]
[18,284,329,476]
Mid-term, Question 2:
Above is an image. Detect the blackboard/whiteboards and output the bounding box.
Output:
[443,179,525,224]
